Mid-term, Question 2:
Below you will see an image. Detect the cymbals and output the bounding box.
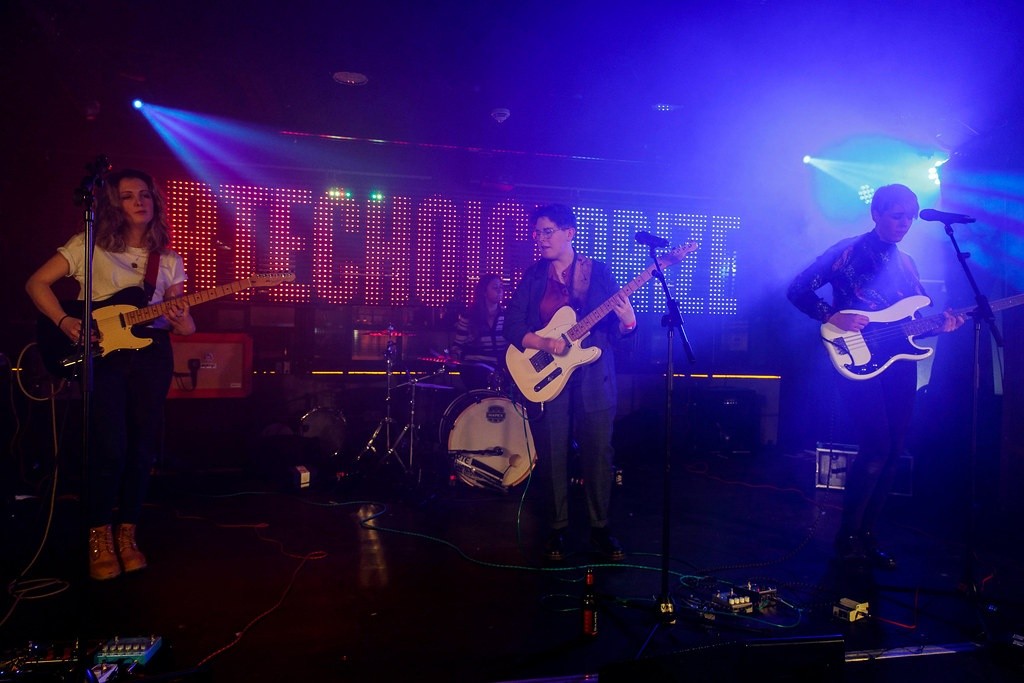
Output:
[417,356,466,365]
[361,330,417,337]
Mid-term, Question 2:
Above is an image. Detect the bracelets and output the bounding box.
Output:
[57,315,68,328]
[623,320,637,330]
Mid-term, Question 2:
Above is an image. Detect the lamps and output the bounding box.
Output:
[329,63,372,86]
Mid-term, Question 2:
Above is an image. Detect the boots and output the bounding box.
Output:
[115,524,147,572]
[88,523,122,579]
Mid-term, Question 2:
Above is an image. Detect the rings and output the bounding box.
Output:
[173,317,177,321]
[553,347,557,353]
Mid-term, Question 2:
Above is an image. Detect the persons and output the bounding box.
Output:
[26,169,197,582]
[781,185,967,570]
[502,203,638,564]
[451,271,510,388]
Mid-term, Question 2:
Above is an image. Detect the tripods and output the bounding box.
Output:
[345,338,446,487]
[630,248,774,665]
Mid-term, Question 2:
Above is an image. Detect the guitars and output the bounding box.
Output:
[505,239,700,402]
[34,269,296,382]
[819,294,1024,382]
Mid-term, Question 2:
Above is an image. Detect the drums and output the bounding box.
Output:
[299,406,347,459]
[399,382,455,431]
[438,389,537,490]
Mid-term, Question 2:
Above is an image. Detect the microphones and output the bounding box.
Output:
[493,446,504,456]
[188,359,200,387]
[95,155,111,168]
[635,231,670,249]
[920,209,977,223]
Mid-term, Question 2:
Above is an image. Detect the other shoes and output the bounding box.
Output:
[835,528,870,562]
[862,527,889,559]
[544,528,569,557]
[591,526,625,558]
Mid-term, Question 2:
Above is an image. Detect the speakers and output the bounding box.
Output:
[166,332,254,398]
[663,372,782,453]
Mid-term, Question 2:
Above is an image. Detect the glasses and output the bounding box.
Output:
[533,228,569,241]
